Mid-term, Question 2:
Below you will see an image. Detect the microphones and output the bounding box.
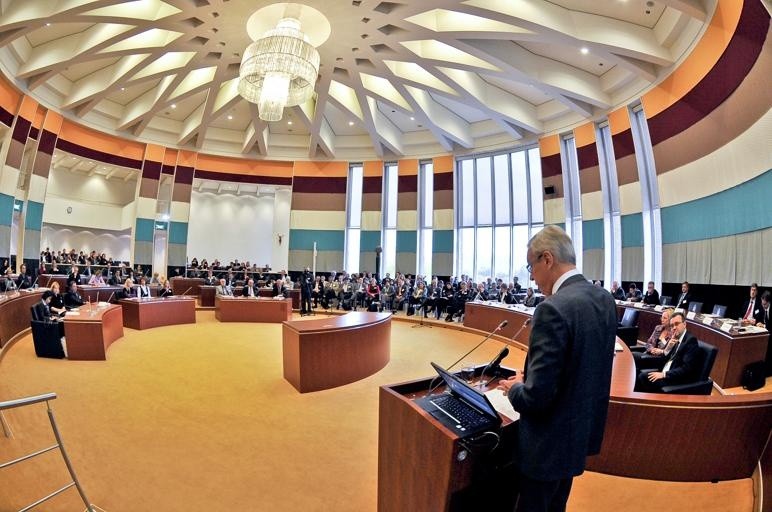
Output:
[477,292,487,305]
[422,320,508,398]
[3,266,290,313]
[512,295,522,310]
[475,318,531,386]
[499,290,506,308]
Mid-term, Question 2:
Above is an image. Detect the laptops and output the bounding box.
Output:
[412,362,502,440]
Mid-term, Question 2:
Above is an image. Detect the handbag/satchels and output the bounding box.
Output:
[742,362,766,391]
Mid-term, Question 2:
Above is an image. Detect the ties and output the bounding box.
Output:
[743,303,753,320]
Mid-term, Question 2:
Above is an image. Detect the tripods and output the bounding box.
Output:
[301,278,316,317]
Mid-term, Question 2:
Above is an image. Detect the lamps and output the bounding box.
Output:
[236,4,331,122]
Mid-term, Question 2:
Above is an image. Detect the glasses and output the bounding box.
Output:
[669,321,682,329]
[527,254,544,274]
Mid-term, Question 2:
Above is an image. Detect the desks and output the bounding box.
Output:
[0,262,772,483]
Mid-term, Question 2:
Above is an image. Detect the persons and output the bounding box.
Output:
[670,282,694,309]
[643,281,661,305]
[635,312,700,394]
[1,248,603,324]
[738,283,764,326]
[609,280,626,300]
[496,225,619,511]
[625,283,643,302]
[631,307,674,369]
[760,291,772,377]
